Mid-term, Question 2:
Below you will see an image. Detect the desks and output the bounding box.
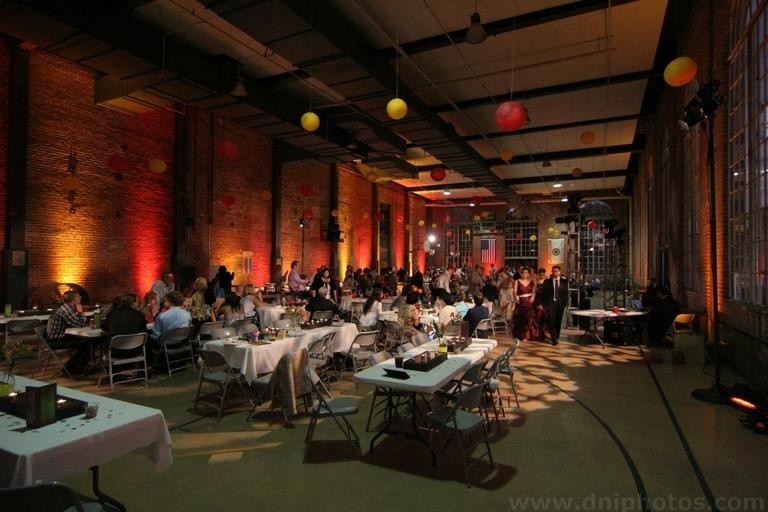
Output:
[353,336,498,467]
[198,324,359,387]
[569,306,647,349]
[65,322,154,337]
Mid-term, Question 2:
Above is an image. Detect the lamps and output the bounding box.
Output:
[442,176,453,197]
[297,209,306,278]
[542,136,553,167]
[467,183,477,208]
[552,160,563,189]
[464,0,485,45]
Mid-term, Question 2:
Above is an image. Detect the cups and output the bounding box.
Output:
[94,313,102,328]
[454,343,460,353]
[4,304,12,317]
[613,306,618,313]
[90,318,94,328]
[394,353,403,367]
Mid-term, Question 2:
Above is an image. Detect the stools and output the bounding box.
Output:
[0,481,86,512]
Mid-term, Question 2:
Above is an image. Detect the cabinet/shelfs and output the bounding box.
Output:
[0,309,102,362]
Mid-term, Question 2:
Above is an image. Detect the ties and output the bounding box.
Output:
[555,280,559,301]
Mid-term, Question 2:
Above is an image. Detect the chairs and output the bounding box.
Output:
[192,321,224,348]
[211,324,258,340]
[664,313,696,343]
[304,364,361,454]
[337,330,379,377]
[194,349,255,423]
[398,344,431,411]
[366,350,411,433]
[425,380,495,480]
[247,348,309,424]
[323,332,337,383]
[35,325,73,378]
[231,317,256,330]
[97,332,147,392]
[439,338,519,433]
[152,326,196,376]
[307,338,333,392]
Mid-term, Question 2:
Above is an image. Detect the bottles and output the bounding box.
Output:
[147,311,152,322]
[247,324,289,342]
